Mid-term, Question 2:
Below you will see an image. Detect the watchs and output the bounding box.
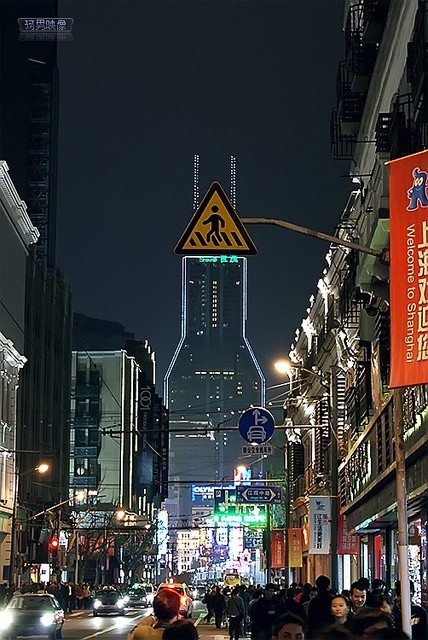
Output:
[151,616,157,626]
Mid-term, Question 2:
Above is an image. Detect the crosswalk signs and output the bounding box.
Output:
[175,182,257,256]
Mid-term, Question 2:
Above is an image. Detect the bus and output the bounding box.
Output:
[224,574,246,586]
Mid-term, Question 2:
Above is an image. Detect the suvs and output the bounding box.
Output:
[155,582,193,619]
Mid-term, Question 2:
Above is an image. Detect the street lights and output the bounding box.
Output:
[270,358,339,590]
[19,493,86,591]
[9,462,49,595]
[237,407,276,444]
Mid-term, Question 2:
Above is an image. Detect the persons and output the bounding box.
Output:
[2,577,134,616]
[328,596,354,627]
[271,614,307,640]
[280,584,289,599]
[357,575,371,592]
[372,579,383,591]
[364,625,411,639]
[299,583,311,603]
[347,577,371,616]
[212,589,225,628]
[133,588,201,639]
[311,575,338,639]
[202,587,218,624]
[393,576,427,638]
[251,584,279,639]
[161,618,199,640]
[212,584,265,597]
[328,587,338,597]
[365,599,395,627]
[226,588,245,639]
[289,582,299,600]
[306,587,318,617]
[320,625,351,640]
[381,585,387,597]
[280,589,307,618]
[386,588,393,599]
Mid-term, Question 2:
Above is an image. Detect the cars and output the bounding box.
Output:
[126,588,147,608]
[93,586,124,616]
[137,583,154,606]
[0,594,64,640]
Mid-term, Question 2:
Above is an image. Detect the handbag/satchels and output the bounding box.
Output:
[230,594,243,620]
